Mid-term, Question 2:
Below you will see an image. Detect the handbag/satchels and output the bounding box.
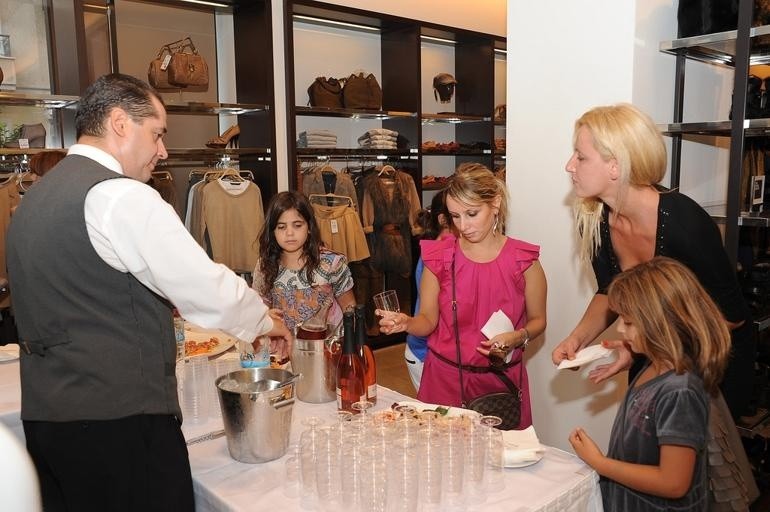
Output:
[168,37,209,87]
[448,239,524,430]
[342,72,383,110]
[307,76,342,109]
[148,45,188,90]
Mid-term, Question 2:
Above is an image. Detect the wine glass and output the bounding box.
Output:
[374,290,408,333]
[286,401,502,512]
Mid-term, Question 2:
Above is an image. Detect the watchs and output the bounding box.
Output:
[516,328,530,351]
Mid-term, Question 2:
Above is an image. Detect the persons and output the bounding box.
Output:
[250,191,357,340]
[404,189,459,393]
[552,103,757,426]
[4,72,293,512]
[568,256,745,512]
[374,161,548,435]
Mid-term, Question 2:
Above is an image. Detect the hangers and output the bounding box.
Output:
[308,183,356,211]
[361,157,396,178]
[9,157,40,191]
[302,154,340,177]
[189,161,252,186]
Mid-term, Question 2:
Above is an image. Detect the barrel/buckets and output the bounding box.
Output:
[214,368,295,464]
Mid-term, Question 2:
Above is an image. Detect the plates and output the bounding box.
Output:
[173,327,235,360]
[490,449,547,467]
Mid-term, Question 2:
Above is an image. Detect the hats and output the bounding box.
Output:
[433,73,457,88]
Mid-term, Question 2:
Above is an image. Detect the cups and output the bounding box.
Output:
[175,353,238,427]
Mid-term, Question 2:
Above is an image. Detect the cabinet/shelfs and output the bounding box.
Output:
[654,0,768,442]
[285,0,507,322]
[0,0,280,341]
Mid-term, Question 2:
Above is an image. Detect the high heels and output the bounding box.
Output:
[205,126,235,148]
[208,124,241,148]
[4,123,47,148]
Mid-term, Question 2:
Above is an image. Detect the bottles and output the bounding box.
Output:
[337,311,366,422]
[295,297,333,340]
[354,303,377,408]
[172,308,185,363]
